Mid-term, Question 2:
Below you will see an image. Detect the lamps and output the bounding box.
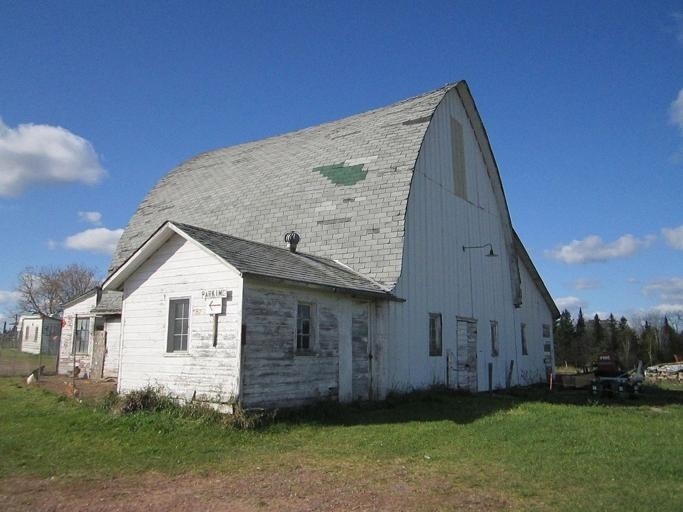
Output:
[462,242,499,258]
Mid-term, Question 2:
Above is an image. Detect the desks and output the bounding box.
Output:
[559,372,594,386]
